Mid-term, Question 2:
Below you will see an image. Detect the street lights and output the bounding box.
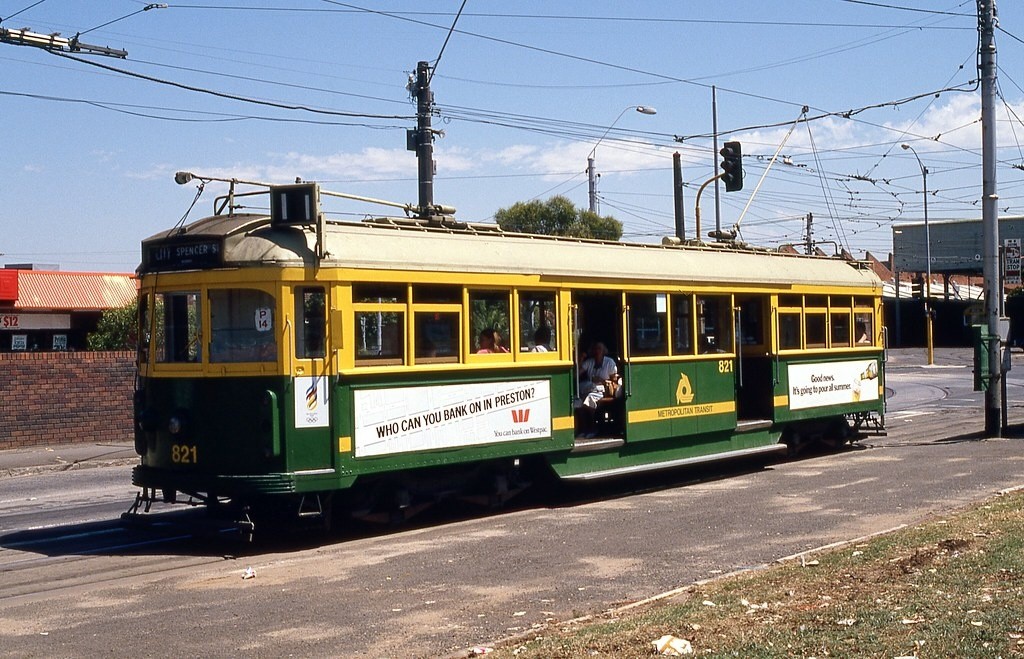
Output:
[900,142,936,365]
[586,105,658,211]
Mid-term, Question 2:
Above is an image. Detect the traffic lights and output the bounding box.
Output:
[911,275,924,299]
[720,141,742,192]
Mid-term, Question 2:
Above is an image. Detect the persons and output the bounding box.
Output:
[530,325,553,352]
[422,338,436,357]
[698,334,711,353]
[575,333,623,438]
[476,328,509,353]
[855,320,870,343]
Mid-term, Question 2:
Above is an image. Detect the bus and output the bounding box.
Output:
[117,106,887,551]
